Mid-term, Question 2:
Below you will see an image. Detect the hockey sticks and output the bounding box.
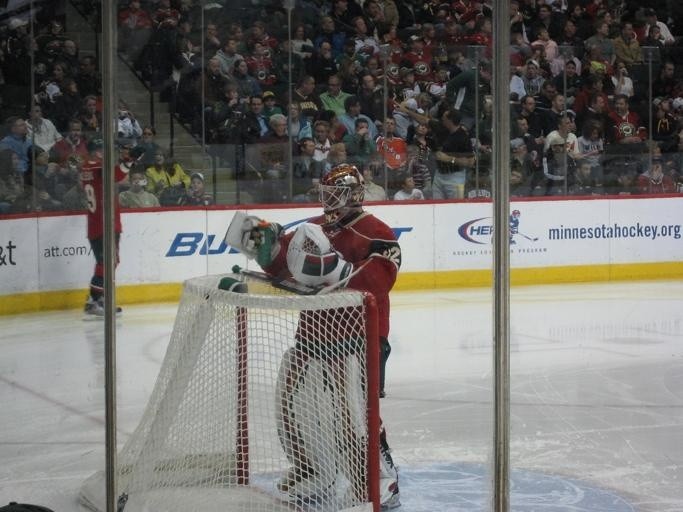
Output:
[231,258,374,295]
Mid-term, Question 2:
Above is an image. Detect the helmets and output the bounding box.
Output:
[319,161,366,226]
[88,138,104,149]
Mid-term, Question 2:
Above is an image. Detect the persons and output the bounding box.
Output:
[0,1,215,215]
[223,162,402,507]
[192,1,496,203]
[505,1,683,197]
[78,134,146,317]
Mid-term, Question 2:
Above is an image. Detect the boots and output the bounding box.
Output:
[84,283,122,315]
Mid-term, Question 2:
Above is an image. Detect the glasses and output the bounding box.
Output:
[143,132,153,136]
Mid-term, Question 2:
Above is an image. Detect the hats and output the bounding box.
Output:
[262,90,275,99]
[191,172,204,181]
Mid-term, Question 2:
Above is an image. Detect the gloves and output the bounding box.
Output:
[242,215,351,289]
[129,145,145,160]
